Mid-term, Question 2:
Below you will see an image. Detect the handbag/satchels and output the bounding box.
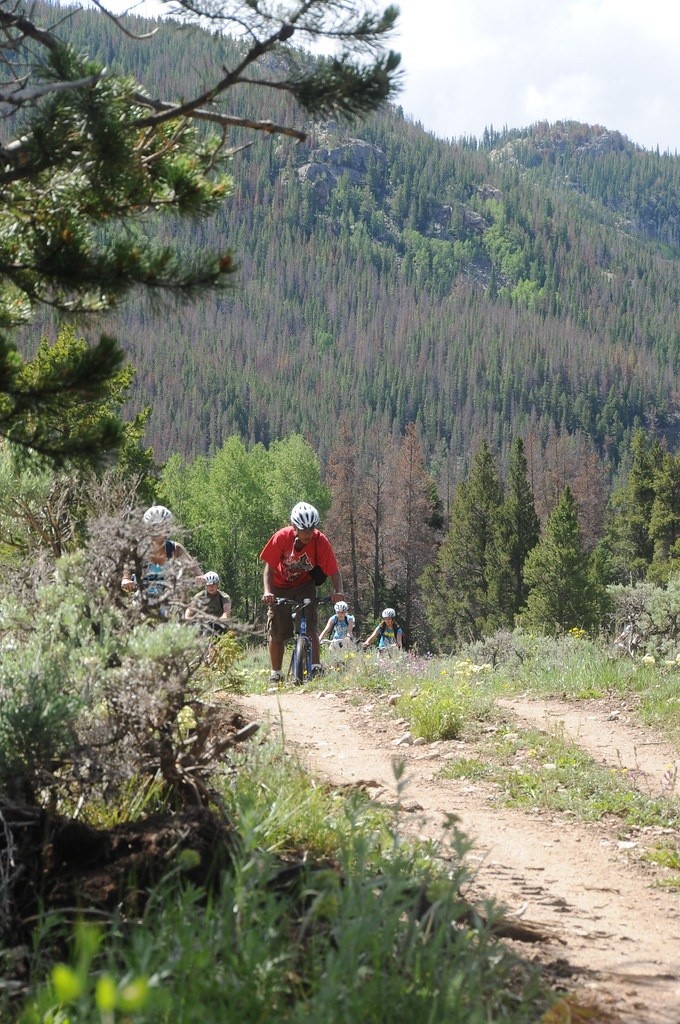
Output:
[309,564,328,587]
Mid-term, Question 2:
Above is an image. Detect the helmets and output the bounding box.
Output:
[291,502,322,529]
[144,506,173,526]
[382,608,396,619]
[333,601,349,613]
[202,571,219,587]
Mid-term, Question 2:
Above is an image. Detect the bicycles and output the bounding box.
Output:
[261,596,332,688]
[318,638,402,678]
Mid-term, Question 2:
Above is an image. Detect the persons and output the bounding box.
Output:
[317,601,355,656]
[364,608,402,660]
[122,505,206,626]
[258,502,344,685]
[185,571,231,645]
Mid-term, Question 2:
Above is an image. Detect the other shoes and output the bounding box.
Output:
[311,667,325,679]
[270,671,283,685]
